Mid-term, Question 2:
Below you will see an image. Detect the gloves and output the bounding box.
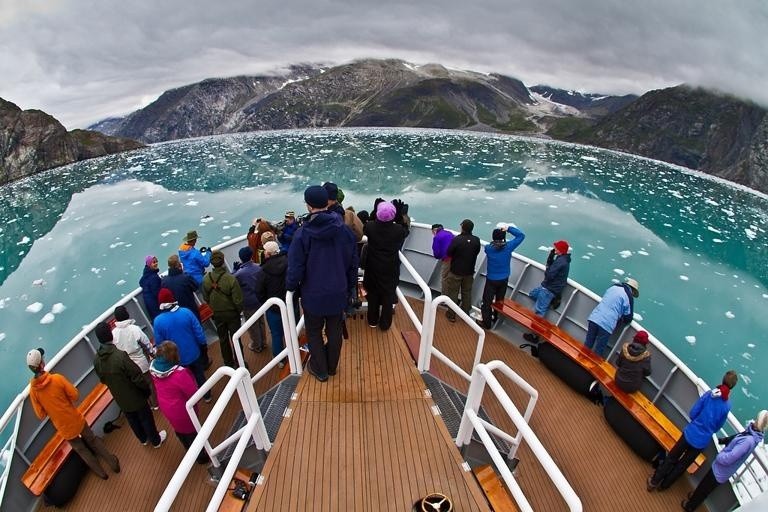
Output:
[200,247,206,253]
[500,225,508,232]
[232,261,242,271]
[393,199,405,217]
[374,198,385,210]
[201,353,209,365]
[206,247,212,252]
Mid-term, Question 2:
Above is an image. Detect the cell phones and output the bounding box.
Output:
[256,219,262,224]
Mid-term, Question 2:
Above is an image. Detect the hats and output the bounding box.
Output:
[757,408,768,432]
[460,220,474,227]
[377,201,397,222]
[158,288,174,303]
[181,231,202,242]
[168,255,179,267]
[622,278,639,298]
[431,224,443,230]
[27,348,45,368]
[492,229,506,239]
[633,331,648,345]
[554,240,569,255]
[239,247,253,261]
[263,241,280,255]
[336,189,344,206]
[211,251,224,264]
[323,182,338,201]
[285,211,294,218]
[96,322,113,343]
[304,185,328,208]
[261,231,275,243]
[145,255,154,266]
[114,306,127,321]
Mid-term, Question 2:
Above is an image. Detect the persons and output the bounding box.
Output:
[681,410,768,512]
[585,279,640,357]
[322,181,410,332]
[286,187,360,382]
[523,241,571,344]
[476,222,525,329]
[445,219,480,322]
[431,224,455,308]
[648,370,738,492]
[615,331,651,394]
[92,211,297,466]
[27,347,120,479]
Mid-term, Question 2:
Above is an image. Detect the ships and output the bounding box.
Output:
[0,215,767,511]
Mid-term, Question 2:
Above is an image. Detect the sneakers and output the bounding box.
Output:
[111,454,120,473]
[552,294,561,310]
[272,355,284,368]
[687,491,694,497]
[247,343,262,352]
[647,473,655,491]
[102,473,109,479]
[445,311,456,322]
[153,402,159,410]
[154,429,167,448]
[141,438,150,446]
[476,319,491,329]
[522,332,538,343]
[307,359,327,382]
[657,481,669,491]
[205,397,213,403]
[681,499,695,511]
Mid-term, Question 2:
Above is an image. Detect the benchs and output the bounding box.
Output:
[490,298,706,475]
[18,301,215,506]
